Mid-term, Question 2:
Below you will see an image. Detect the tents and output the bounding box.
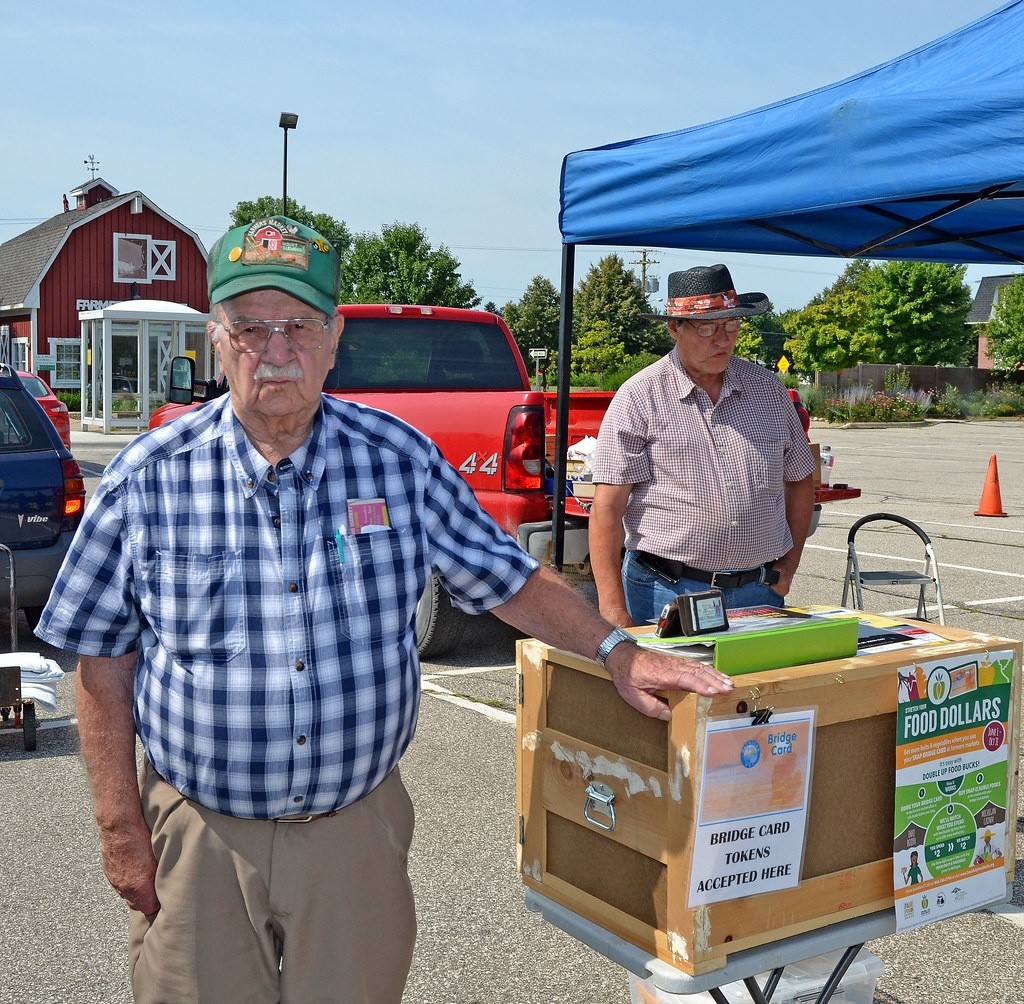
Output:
[551,0,1024,573]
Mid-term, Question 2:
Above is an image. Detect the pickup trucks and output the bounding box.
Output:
[148,304,809,662]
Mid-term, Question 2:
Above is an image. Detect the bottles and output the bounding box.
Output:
[820,446,834,484]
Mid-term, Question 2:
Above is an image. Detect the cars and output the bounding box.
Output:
[13,370,71,456]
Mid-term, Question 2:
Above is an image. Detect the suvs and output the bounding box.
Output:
[0,363,88,637]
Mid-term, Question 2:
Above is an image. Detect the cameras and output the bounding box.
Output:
[655,603,676,637]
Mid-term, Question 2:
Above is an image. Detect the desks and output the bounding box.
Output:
[525,883,1012,1004]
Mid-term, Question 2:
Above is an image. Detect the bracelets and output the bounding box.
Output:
[596,628,637,669]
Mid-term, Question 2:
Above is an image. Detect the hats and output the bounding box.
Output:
[637,263,770,319]
[787,388,801,402]
[205,214,342,317]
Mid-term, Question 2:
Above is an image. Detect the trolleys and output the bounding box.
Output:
[1,544,39,751]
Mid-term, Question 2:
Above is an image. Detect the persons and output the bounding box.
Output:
[33,216,736,1004]
[589,263,817,629]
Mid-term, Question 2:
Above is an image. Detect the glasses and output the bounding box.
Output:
[213,313,337,353]
[685,319,741,337]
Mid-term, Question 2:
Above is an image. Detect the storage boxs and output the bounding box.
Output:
[546,434,595,497]
[808,443,821,489]
[516,605,1023,976]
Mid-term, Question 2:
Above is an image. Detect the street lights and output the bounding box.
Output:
[279,112,299,221]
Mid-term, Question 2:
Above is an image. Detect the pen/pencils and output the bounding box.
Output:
[334,528,345,564]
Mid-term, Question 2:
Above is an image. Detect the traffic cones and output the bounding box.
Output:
[974,453,1009,517]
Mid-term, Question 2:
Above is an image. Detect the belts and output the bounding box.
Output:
[274,810,338,823]
[635,549,780,589]
